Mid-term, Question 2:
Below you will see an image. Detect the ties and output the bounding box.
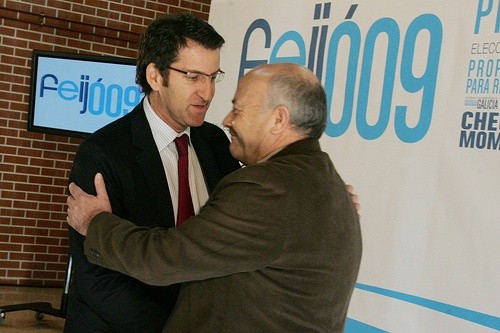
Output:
[175,134,197,232]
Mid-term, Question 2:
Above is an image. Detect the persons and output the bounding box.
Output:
[62,12,362,333]
[65,61,363,333]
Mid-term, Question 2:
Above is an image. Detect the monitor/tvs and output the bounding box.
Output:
[26,49,144,136]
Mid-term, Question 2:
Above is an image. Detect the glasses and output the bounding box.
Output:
[157,65,225,84]
